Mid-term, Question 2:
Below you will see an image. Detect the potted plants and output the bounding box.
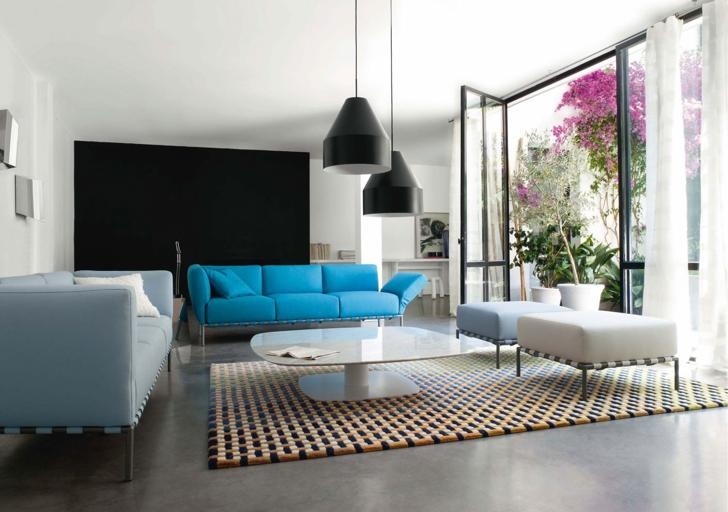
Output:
[507,127,605,312]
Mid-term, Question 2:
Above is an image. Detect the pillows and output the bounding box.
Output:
[208,268,256,299]
[73,272,161,319]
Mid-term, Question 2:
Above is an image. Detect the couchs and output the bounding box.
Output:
[187,263,428,346]
[0,269,173,483]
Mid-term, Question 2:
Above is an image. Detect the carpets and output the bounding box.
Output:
[207,346,728,471]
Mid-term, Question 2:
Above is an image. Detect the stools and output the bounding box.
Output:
[456,300,680,401]
[417,276,444,299]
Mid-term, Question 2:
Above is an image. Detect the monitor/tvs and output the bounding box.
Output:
[73,140,310,299]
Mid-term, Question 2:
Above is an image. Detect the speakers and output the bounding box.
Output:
[0,109,19,169]
[16,175,40,219]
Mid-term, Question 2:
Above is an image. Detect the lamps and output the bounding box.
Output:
[322,0,425,218]
